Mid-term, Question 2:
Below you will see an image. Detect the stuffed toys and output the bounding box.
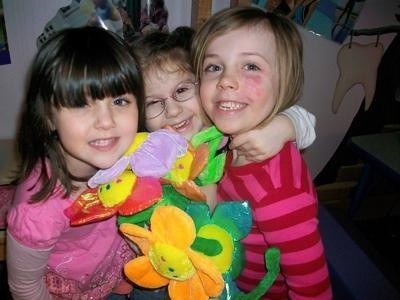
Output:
[66,127,280,300]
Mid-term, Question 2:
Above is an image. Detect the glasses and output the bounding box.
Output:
[142,78,201,120]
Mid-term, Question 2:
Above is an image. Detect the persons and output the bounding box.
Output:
[128,27,317,299]
[6,25,144,299]
[189,5,335,300]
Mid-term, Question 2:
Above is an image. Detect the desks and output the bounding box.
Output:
[346,130,400,221]
